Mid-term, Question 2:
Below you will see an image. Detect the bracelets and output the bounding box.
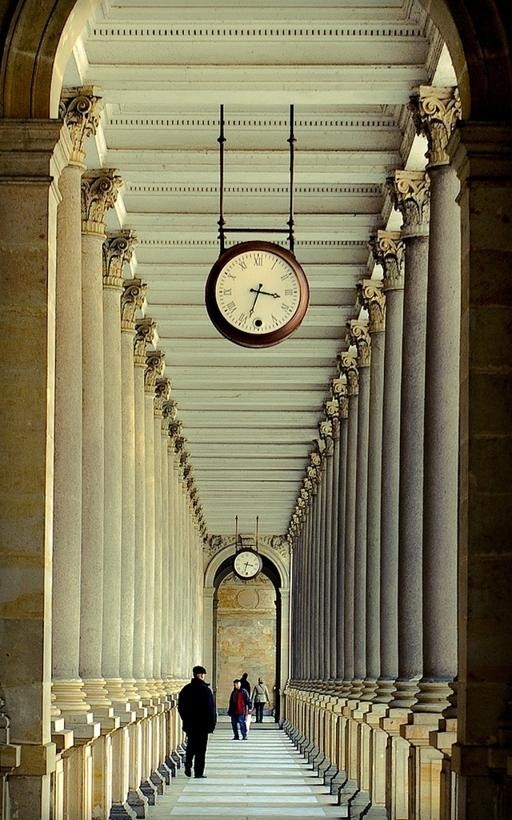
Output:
[248,709,252,712]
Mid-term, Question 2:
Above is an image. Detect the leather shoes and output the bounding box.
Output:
[184,762,206,778]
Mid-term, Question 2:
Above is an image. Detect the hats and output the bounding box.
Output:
[193,666,206,674]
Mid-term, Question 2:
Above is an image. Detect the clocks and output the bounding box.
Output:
[204,104,310,350]
[231,516,265,581]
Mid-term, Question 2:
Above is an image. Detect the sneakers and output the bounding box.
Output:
[233,736,248,740]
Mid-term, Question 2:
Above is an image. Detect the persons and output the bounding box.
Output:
[240,672,251,698]
[176,664,218,779]
[242,687,254,736]
[251,677,272,723]
[227,678,253,741]
